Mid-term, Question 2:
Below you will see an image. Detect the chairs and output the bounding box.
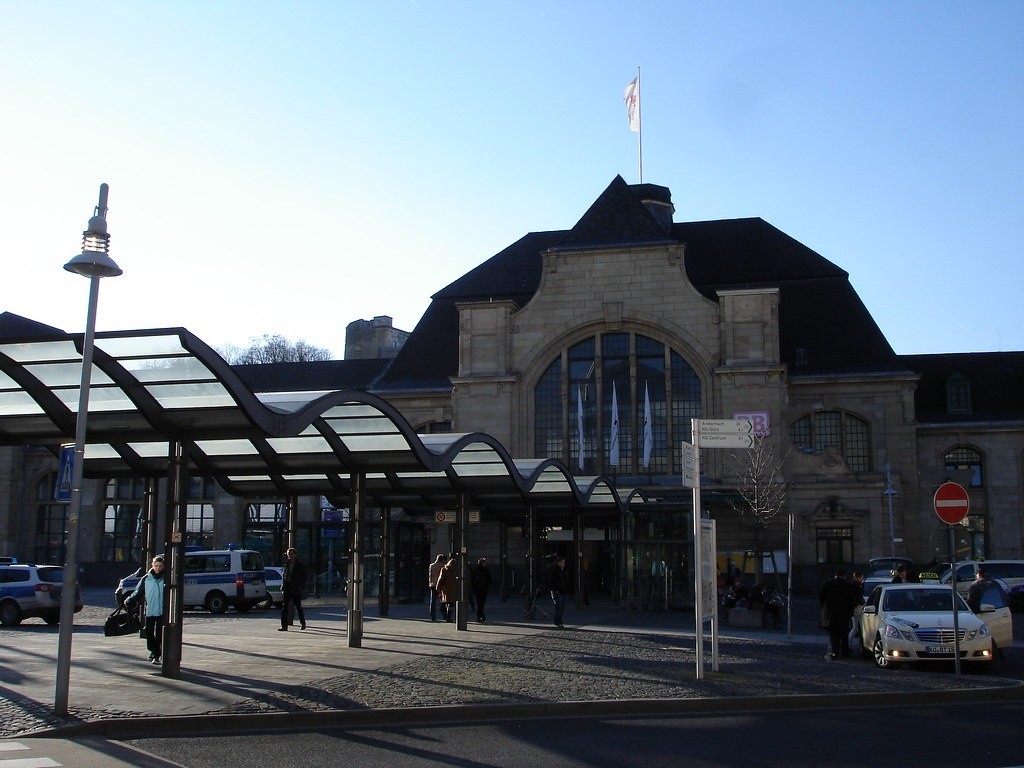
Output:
[188,559,200,573]
[886,595,936,611]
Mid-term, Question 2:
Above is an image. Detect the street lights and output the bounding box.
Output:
[52,179,125,716]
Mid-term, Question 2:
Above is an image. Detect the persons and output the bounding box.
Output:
[819,570,867,662]
[967,569,1004,662]
[428,554,457,623]
[279,548,307,631]
[716,572,788,630]
[124,557,165,664]
[468,558,488,623]
[549,558,566,628]
[892,564,923,600]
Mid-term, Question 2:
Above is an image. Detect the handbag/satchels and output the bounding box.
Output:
[105,604,141,636]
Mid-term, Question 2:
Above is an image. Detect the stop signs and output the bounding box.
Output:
[935,482,969,523]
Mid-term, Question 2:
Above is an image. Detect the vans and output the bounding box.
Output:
[116,543,269,616]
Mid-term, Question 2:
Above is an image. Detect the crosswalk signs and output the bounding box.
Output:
[54,443,75,502]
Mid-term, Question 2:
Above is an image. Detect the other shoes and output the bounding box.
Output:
[301,623,307,630]
[430,617,436,620]
[446,617,453,623]
[476,618,482,623]
[278,626,288,631]
[831,652,840,661]
[154,657,161,665]
[149,653,154,661]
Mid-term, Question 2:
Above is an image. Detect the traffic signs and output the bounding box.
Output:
[698,434,755,449]
[697,419,754,434]
[682,441,697,488]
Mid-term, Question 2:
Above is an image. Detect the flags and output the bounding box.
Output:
[623,77,640,132]
[577,390,584,470]
[644,389,652,467]
[610,384,618,465]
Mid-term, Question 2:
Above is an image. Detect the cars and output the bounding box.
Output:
[0,557,84,628]
[850,556,1024,672]
[254,567,287,610]
